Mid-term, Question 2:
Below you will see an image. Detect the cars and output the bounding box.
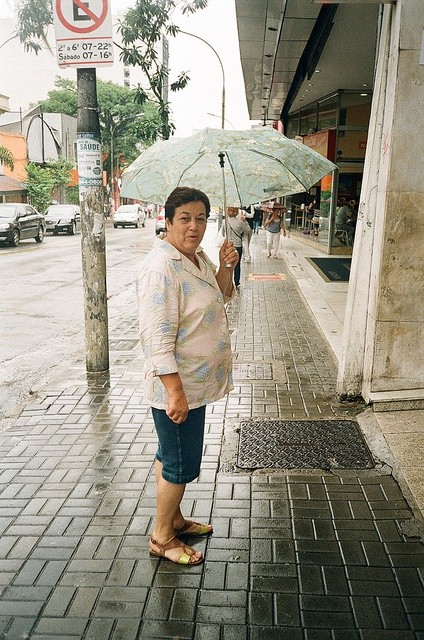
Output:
[207,209,217,222]
[44,205,81,235]
[0,203,45,247]
[156,208,167,235]
[114,204,146,228]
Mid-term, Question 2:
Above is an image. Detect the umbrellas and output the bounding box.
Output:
[119,128,340,268]
[259,202,288,215]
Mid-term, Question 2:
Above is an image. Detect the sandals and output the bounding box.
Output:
[172,520,213,536]
[149,536,204,565]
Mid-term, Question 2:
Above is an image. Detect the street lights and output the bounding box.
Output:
[208,113,235,131]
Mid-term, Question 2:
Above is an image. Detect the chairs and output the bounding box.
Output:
[312,210,320,234]
[304,206,313,232]
[333,223,349,244]
[295,208,304,227]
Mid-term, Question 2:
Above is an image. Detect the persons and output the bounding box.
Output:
[335,203,354,246]
[306,197,321,236]
[253,203,263,234]
[264,209,286,259]
[235,205,256,263]
[222,207,252,290]
[136,187,239,565]
